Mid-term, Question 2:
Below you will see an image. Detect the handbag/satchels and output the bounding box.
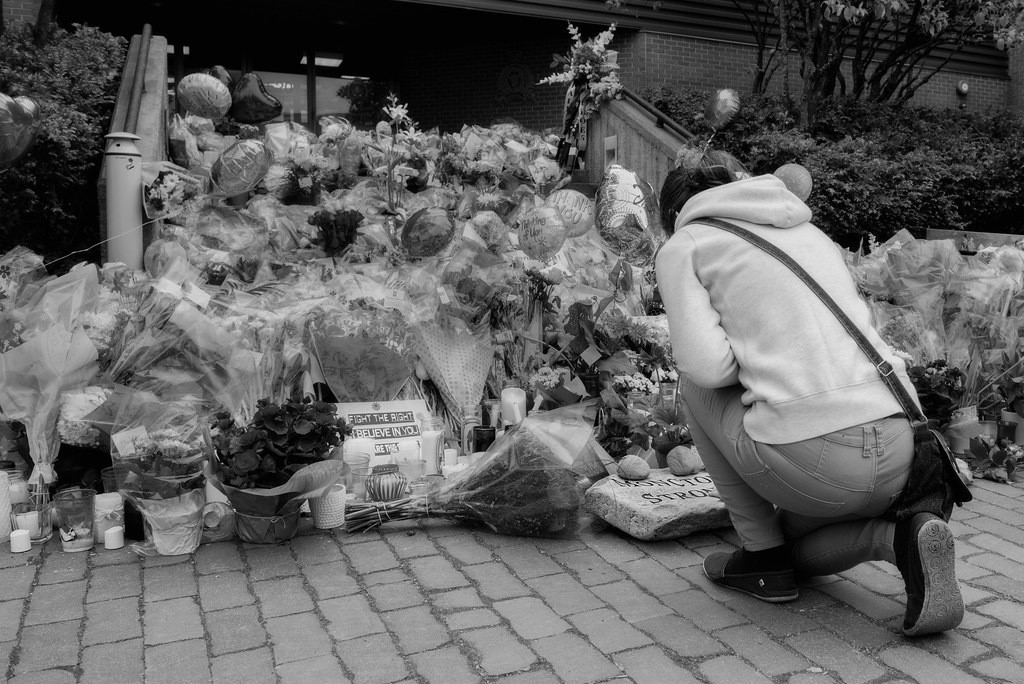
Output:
[901,428,974,504]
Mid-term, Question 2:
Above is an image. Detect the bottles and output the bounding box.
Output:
[420,416,444,476]
[365,464,407,500]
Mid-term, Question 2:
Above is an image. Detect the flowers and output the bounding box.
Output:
[0,22,1024,538]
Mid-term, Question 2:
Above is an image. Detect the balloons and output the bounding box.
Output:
[703,87,741,130]
[174,64,662,265]
[771,163,814,203]
[0,93,41,168]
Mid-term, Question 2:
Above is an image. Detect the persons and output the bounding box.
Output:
[653,160,965,638]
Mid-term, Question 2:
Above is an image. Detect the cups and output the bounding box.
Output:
[53,488,96,553]
[342,451,373,502]
[396,459,446,500]
[9,502,53,546]
[472,426,496,452]
[471,452,485,464]
[101,467,118,493]
[309,484,346,529]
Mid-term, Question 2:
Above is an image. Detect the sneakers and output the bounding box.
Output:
[902,512,964,638]
[702,550,800,603]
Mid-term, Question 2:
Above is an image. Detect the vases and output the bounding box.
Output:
[305,387,526,528]
[575,372,599,399]
[101,466,144,541]
[949,383,1024,454]
[54,490,96,552]
[655,443,696,469]
[95,494,124,543]
[149,507,204,554]
[10,504,53,543]
